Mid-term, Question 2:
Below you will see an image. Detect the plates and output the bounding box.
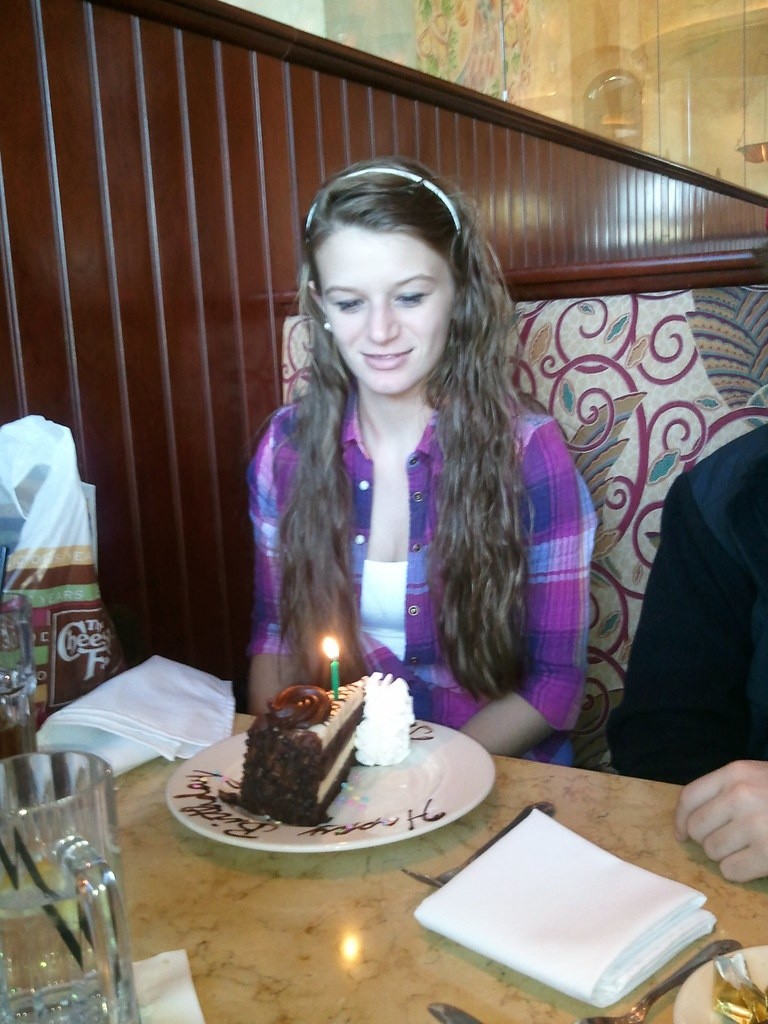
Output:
[672,943,768,1023]
[164,718,498,855]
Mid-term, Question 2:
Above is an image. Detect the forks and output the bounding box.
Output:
[400,800,558,888]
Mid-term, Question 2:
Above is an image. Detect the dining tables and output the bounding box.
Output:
[0,710,767,1022]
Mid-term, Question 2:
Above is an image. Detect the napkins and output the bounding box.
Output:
[127,950,206,1024]
[414,809,716,1009]
[37,652,234,782]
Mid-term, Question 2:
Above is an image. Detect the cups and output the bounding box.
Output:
[1,748,140,1023]
[1,587,42,754]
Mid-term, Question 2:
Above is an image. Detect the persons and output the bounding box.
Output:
[606,421,767,882]
[246,156,602,759]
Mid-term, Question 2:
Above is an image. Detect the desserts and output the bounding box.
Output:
[239,676,370,828]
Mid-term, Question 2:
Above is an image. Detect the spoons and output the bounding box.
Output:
[574,937,742,1024]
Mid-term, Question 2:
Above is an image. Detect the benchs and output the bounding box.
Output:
[280,239,767,775]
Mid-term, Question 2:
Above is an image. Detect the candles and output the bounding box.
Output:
[323,640,340,701]
